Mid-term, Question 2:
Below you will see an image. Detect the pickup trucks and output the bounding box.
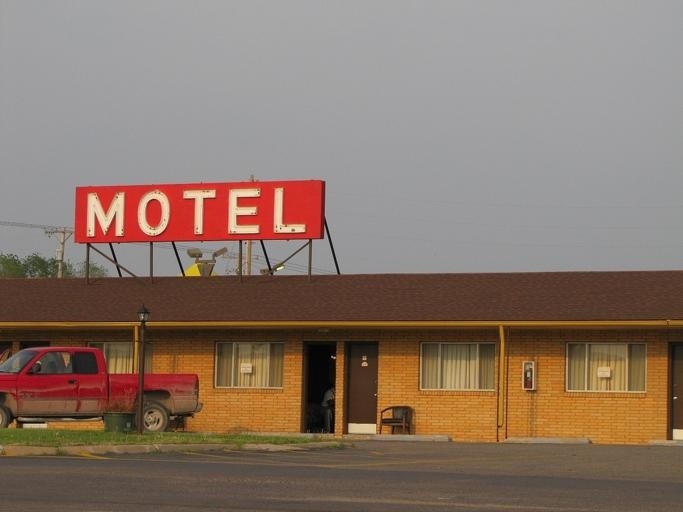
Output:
[0,346,202,430]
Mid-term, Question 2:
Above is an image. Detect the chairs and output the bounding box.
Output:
[379,406,412,435]
